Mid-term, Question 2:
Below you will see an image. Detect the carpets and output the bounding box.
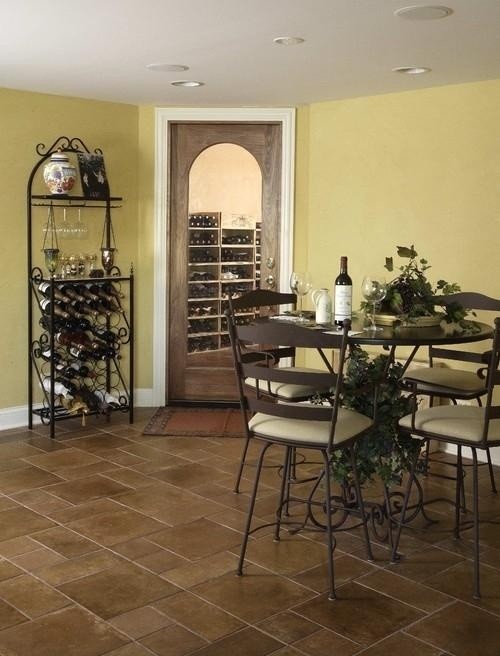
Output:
[141,405,257,439]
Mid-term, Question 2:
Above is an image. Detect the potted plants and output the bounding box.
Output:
[358,244,481,335]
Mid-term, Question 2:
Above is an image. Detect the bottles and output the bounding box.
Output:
[188,213,219,351]
[220,229,255,343]
[60,253,96,277]
[256,221,261,292]
[38,283,127,403]
[311,288,332,325]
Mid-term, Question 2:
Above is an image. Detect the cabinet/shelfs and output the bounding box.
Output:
[185,210,264,357]
[25,136,136,439]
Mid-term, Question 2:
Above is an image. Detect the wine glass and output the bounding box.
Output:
[73,206,89,242]
[360,273,388,334]
[289,271,312,324]
[41,207,58,241]
[57,206,73,240]
[333,256,354,327]
